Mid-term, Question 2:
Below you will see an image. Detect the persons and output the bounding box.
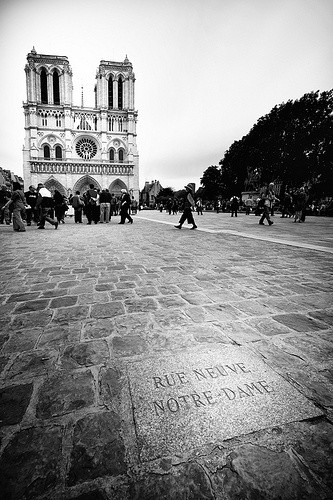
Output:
[1,182,27,231]
[174,184,198,230]
[34,182,59,230]
[0,177,138,225]
[140,188,333,220]
[258,183,280,226]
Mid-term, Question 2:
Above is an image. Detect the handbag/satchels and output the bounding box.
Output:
[186,193,196,206]
[257,199,266,207]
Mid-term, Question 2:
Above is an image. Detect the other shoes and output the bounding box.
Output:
[54,222,58,229]
[75,220,110,225]
[189,225,197,230]
[38,226,45,229]
[111,212,121,216]
[174,225,181,229]
[118,222,125,224]
[14,229,26,232]
[259,222,264,225]
[131,219,133,224]
[37,222,40,226]
[280,215,305,223]
[0,220,31,226]
[269,222,273,225]
[271,215,275,216]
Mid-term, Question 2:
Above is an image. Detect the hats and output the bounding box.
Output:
[184,183,193,191]
